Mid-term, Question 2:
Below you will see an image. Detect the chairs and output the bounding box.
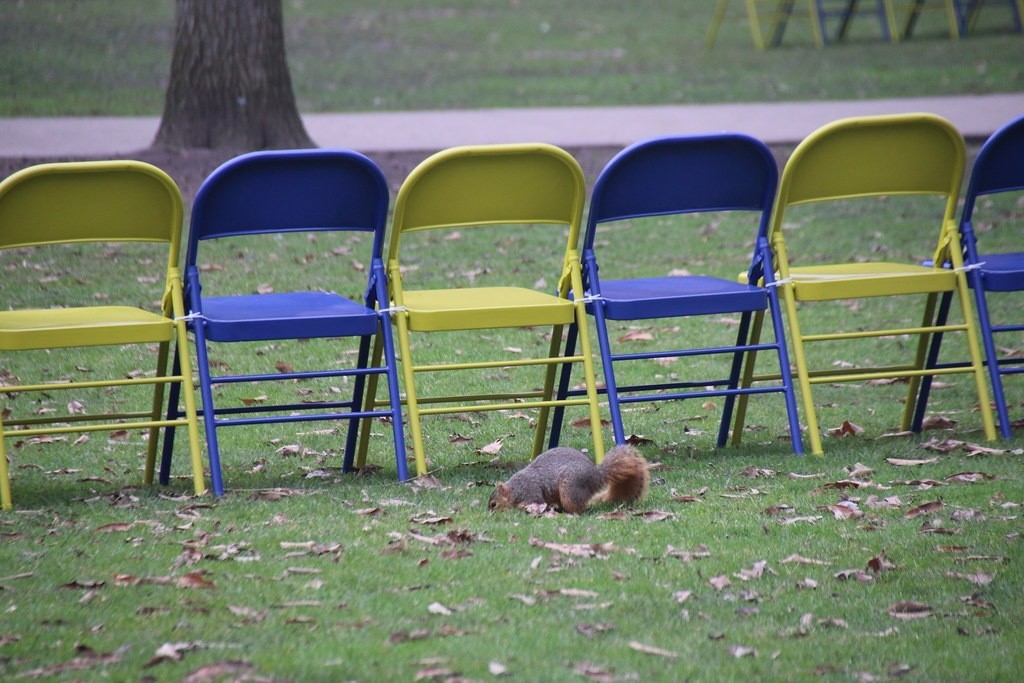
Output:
[730,114,997,457]
[548,131,803,459]
[0,159,206,509]
[357,144,604,479]
[911,116,1024,442]
[159,148,410,496]
